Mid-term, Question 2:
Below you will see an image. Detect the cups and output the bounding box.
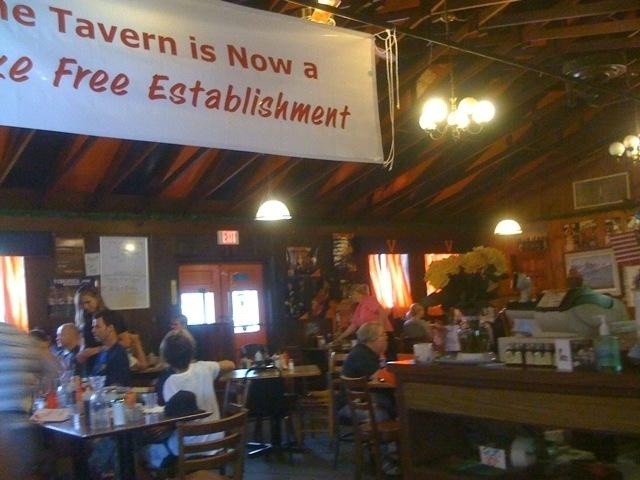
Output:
[412,342,433,365]
[141,392,159,409]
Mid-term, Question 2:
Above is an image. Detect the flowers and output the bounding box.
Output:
[416,242,516,352]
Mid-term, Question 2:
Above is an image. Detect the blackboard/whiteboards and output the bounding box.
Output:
[99,236,149,310]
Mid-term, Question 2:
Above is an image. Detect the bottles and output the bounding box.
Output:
[254,350,294,372]
[47,375,144,434]
[509,427,538,474]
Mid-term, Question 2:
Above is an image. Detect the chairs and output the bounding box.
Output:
[1,302,640,479]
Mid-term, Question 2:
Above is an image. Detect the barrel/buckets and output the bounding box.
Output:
[498,433,537,466]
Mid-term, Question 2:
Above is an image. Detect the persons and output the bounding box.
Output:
[0,284,640,480]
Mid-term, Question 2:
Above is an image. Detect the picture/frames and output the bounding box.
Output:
[562,247,623,298]
[570,170,632,211]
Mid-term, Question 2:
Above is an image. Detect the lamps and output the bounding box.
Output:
[254,188,292,223]
[415,9,500,142]
[606,129,640,165]
